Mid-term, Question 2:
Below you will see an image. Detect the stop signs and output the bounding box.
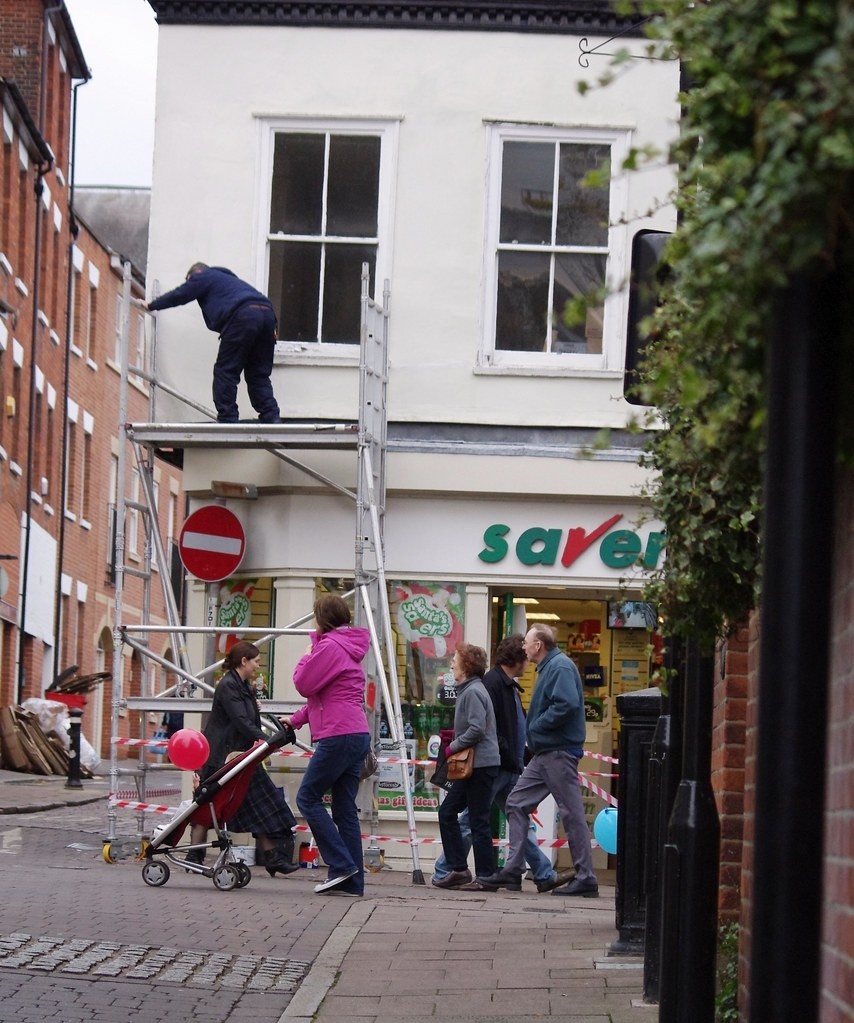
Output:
[181,507,250,584]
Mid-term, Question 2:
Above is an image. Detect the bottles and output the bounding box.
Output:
[403,723,413,739]
[380,722,387,738]
[417,713,451,740]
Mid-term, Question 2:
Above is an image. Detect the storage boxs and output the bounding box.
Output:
[299,844,319,867]
[377,702,454,791]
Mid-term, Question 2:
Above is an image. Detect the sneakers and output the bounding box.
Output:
[314,869,361,897]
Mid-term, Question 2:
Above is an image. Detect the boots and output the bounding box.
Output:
[264,848,299,877]
[185,850,204,874]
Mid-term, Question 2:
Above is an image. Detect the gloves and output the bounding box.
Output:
[440,729,454,741]
[446,746,452,757]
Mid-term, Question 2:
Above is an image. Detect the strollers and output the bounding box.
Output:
[139,711,297,888]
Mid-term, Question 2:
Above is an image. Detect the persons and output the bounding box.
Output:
[433,641,501,892]
[162,694,184,763]
[279,594,371,896]
[431,634,577,894]
[476,623,599,897]
[137,262,281,425]
[184,642,302,877]
[569,634,599,650]
[152,751,243,839]
[610,603,655,627]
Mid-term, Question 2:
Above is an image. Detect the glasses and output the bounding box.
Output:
[522,640,538,645]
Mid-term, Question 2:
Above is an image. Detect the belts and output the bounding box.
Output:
[250,304,270,309]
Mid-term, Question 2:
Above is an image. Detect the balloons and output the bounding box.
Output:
[169,728,210,770]
[594,808,618,854]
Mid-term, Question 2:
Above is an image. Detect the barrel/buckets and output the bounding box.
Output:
[230,844,256,867]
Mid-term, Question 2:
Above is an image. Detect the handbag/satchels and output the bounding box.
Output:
[362,745,378,779]
[430,739,456,792]
[447,748,474,780]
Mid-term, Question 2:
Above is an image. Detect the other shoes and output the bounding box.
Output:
[537,871,576,892]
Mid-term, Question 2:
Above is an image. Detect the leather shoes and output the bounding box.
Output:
[432,869,472,887]
[551,878,599,897]
[460,879,497,892]
[476,870,522,891]
